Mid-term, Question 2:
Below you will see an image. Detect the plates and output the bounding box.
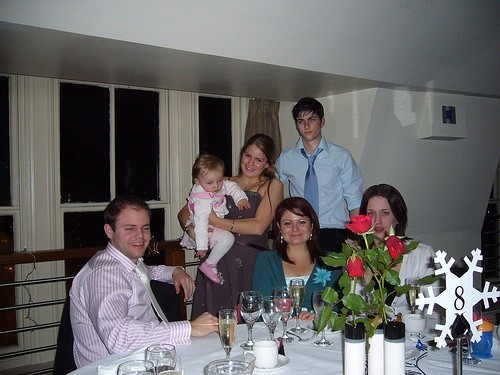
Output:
[307,322,345,334]
[254,354,290,372]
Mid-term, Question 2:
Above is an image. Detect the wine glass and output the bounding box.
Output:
[289,279,308,335]
[261,296,283,341]
[273,286,297,343]
[219,309,237,359]
[312,290,333,347]
[405,277,421,314]
[239,290,262,350]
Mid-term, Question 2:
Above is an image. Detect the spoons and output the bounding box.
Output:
[286,330,309,342]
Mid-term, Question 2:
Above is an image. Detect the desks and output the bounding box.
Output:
[66,320,500,375]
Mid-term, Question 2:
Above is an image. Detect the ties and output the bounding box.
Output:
[300,148,323,219]
[134,263,168,324]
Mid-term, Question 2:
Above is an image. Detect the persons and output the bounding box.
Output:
[251,197,342,323]
[69,194,219,369]
[349,184,440,333]
[179,154,251,284]
[178,134,284,321]
[275,98,363,254]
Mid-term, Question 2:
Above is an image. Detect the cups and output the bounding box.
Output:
[243,341,278,369]
[470,331,493,359]
[116,343,183,375]
[425,312,442,329]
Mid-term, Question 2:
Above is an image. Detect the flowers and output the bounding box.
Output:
[318,215,444,355]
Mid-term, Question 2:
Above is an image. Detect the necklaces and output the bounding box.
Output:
[292,261,311,276]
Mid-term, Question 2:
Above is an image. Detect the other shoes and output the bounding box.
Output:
[199,260,222,283]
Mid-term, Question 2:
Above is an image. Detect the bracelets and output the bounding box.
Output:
[230,218,234,232]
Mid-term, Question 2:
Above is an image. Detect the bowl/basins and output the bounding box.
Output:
[204,359,253,375]
[403,313,428,333]
